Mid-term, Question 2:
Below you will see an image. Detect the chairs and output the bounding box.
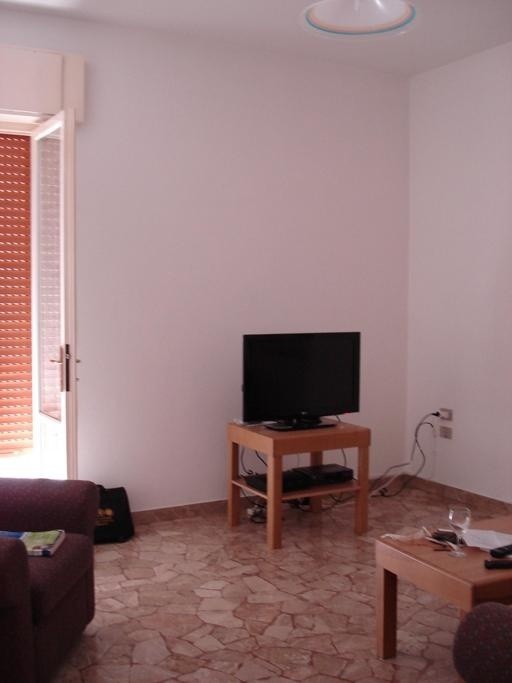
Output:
[0,478,101,683]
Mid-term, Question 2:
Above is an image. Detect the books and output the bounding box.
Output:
[0,528,67,556]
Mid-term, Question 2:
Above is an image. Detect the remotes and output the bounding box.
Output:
[484,559,512,571]
[490,544,512,558]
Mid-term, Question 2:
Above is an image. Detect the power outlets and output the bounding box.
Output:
[436,409,451,420]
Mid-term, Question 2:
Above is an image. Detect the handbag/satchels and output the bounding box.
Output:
[92,485,134,543]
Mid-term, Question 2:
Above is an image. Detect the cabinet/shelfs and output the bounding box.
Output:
[225,422,372,550]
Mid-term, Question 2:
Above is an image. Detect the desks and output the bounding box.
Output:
[374,513,512,658]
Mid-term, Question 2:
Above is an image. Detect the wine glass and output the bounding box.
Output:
[446,507,469,561]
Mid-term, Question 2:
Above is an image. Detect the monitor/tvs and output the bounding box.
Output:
[242,332,360,431]
